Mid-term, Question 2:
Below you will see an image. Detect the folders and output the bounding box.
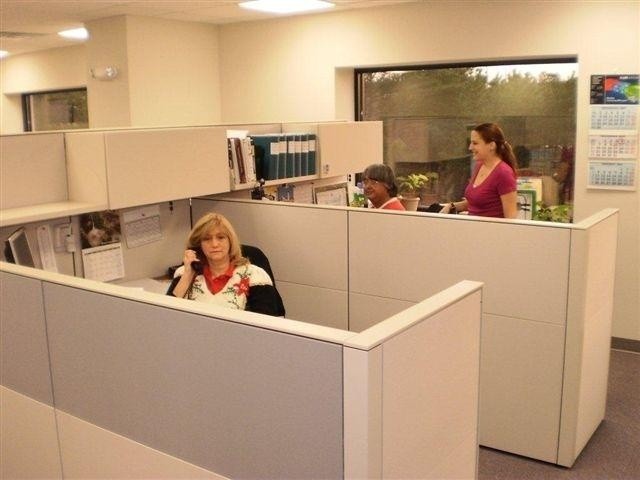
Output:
[247,133,316,180]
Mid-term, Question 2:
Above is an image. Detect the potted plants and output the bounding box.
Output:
[396,174,428,211]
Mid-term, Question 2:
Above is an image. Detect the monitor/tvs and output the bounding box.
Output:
[4,227,34,267]
[312,183,349,207]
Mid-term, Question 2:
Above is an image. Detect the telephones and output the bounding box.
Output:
[188,247,205,275]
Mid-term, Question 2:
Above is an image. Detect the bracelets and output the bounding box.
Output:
[451,202,456,213]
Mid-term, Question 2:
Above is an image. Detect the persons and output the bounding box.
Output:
[427,122,520,219]
[360,163,407,211]
[164,212,287,318]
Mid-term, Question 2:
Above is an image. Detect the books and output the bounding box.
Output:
[227,131,316,185]
